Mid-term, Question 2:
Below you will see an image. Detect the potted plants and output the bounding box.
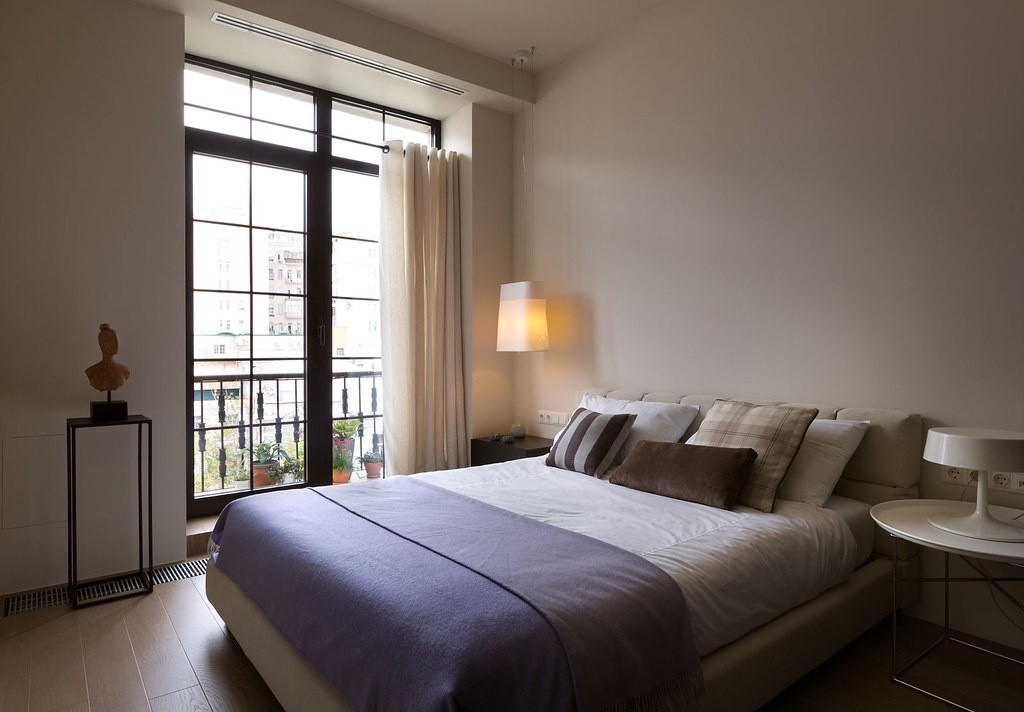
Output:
[232,469,249,489]
[332,450,354,483]
[333,419,366,457]
[356,450,384,477]
[240,439,292,486]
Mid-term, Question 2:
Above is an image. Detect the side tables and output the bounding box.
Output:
[869,498,1024,712]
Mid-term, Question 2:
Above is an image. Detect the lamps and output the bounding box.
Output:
[922,427,1024,543]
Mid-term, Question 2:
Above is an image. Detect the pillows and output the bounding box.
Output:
[609,440,757,511]
[546,408,637,478]
[686,419,870,508]
[691,399,820,512]
[550,392,700,452]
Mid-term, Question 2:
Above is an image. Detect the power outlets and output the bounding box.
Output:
[538,410,546,423]
[546,411,553,424]
[942,465,1024,494]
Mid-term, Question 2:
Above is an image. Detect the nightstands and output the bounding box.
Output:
[470,434,554,467]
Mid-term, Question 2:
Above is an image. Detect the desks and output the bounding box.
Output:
[66,413,152,603]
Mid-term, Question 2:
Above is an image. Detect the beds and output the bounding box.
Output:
[205,407,921,712]
